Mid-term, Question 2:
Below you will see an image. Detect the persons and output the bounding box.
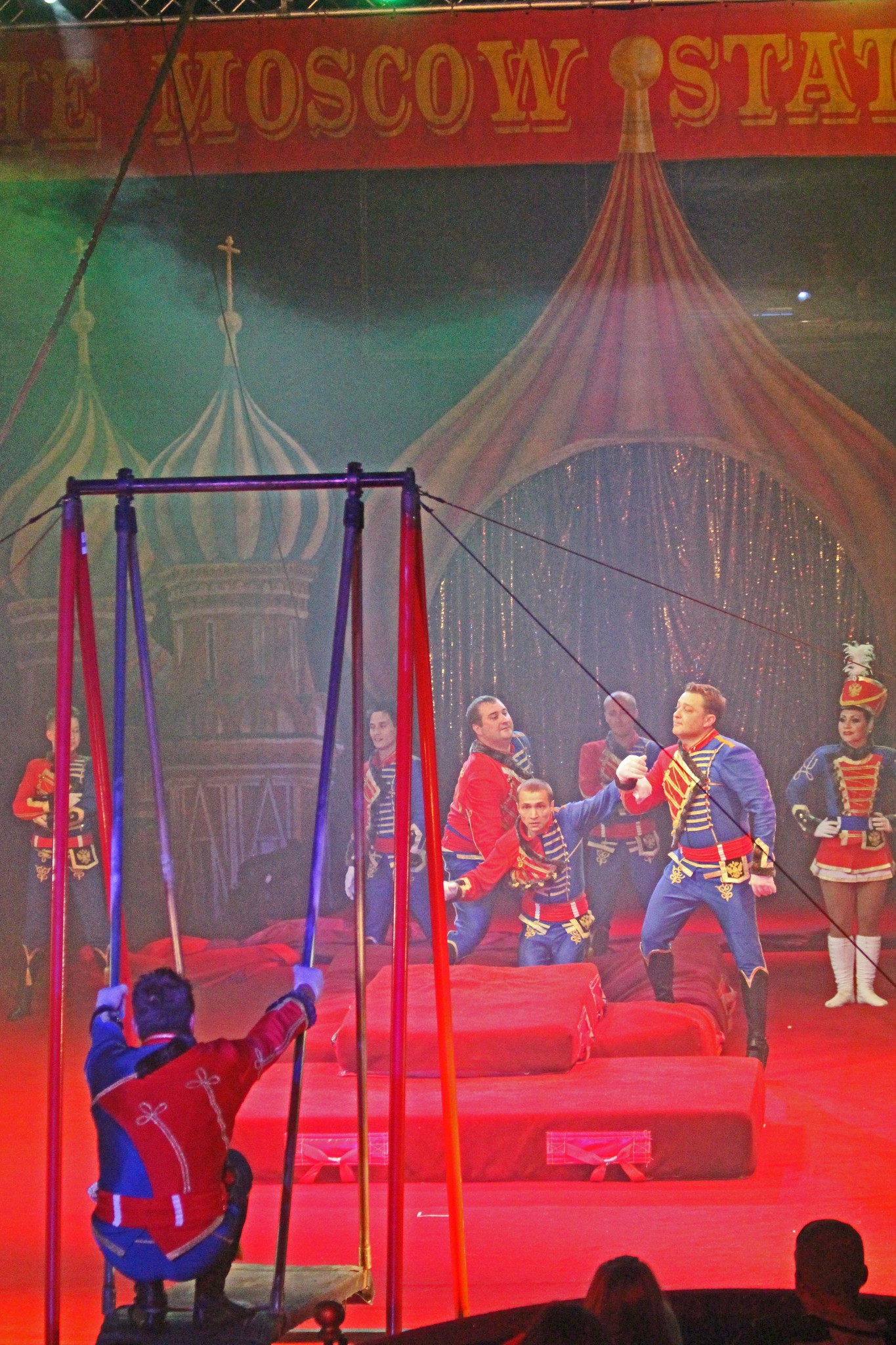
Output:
[7,700,123,1022]
[729,1218,895,1345]
[85,963,325,1333]
[342,700,433,944]
[426,694,542,971]
[615,680,778,1070]
[443,774,654,967]
[579,689,674,958]
[497,1303,610,1345]
[783,676,896,1007]
[578,1256,684,1345]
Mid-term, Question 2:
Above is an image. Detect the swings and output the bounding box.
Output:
[96,502,373,1344]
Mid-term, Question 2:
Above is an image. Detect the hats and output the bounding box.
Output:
[838,676,889,720]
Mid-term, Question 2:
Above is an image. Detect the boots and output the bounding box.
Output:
[825,933,856,1008]
[737,966,769,1074]
[855,935,888,1009]
[185,1184,256,1336]
[88,943,114,1008]
[637,942,676,1006]
[587,922,612,957]
[126,1279,168,1331]
[7,941,45,1021]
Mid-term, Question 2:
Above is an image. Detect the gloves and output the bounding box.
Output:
[344,865,356,901]
[94,981,128,1020]
[871,812,894,833]
[634,776,654,801]
[615,754,648,785]
[748,875,778,899]
[68,792,82,809]
[442,879,459,903]
[292,964,324,1003]
[812,817,839,839]
